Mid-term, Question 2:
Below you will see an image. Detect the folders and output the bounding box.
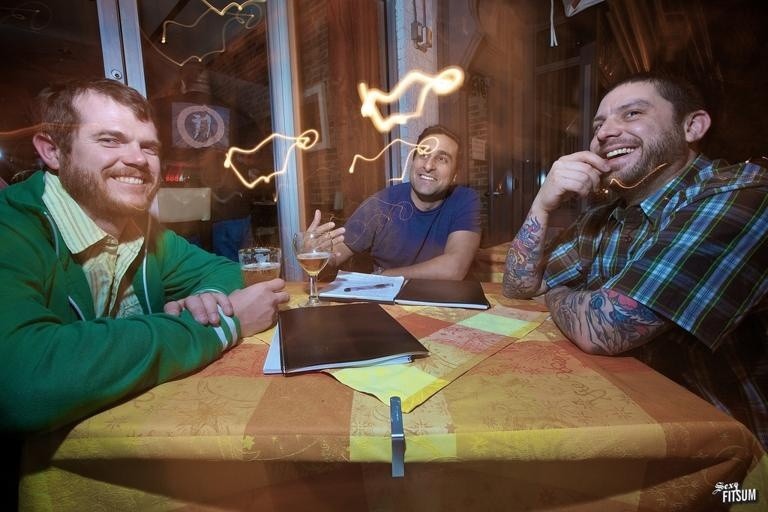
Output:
[393,278,491,310]
[277,302,429,374]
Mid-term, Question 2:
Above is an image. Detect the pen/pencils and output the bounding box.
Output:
[344,283,392,293]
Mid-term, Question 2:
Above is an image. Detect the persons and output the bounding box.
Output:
[202,138,256,263]
[0,76,291,511]
[501,74,768,510]
[300,125,483,285]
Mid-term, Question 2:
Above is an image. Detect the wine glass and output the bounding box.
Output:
[291,231,333,308]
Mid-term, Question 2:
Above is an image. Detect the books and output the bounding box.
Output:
[263,269,494,375]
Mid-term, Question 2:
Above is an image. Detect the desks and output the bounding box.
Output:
[53,270,756,511]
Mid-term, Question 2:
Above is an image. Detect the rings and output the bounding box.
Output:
[308,233,315,240]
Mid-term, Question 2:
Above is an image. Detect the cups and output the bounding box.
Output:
[237,248,282,289]
